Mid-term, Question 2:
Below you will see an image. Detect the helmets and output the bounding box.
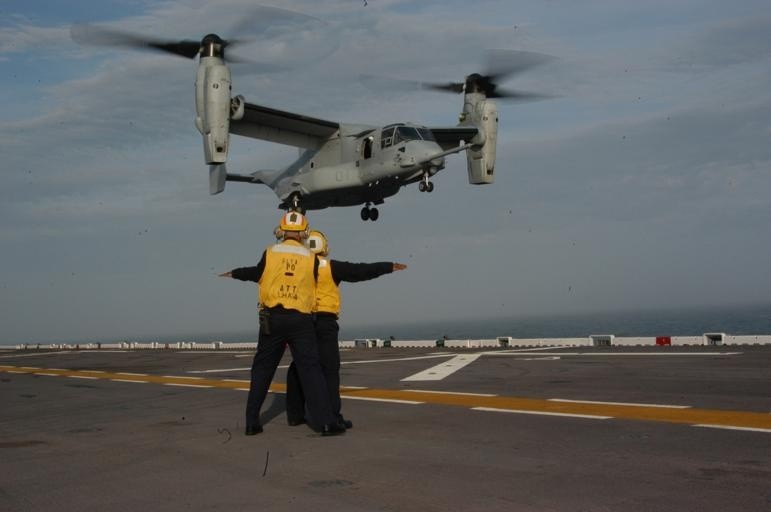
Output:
[275,211,329,257]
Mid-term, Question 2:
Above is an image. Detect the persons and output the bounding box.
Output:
[245,212,344,436]
[219,231,406,428]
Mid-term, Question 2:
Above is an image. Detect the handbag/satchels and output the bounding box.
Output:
[259,311,272,334]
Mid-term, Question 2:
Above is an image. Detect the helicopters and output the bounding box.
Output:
[74,8,563,221]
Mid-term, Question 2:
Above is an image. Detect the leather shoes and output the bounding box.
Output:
[287,415,352,435]
[245,425,263,436]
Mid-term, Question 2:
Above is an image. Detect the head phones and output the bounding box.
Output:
[274,211,310,239]
[311,231,329,256]
